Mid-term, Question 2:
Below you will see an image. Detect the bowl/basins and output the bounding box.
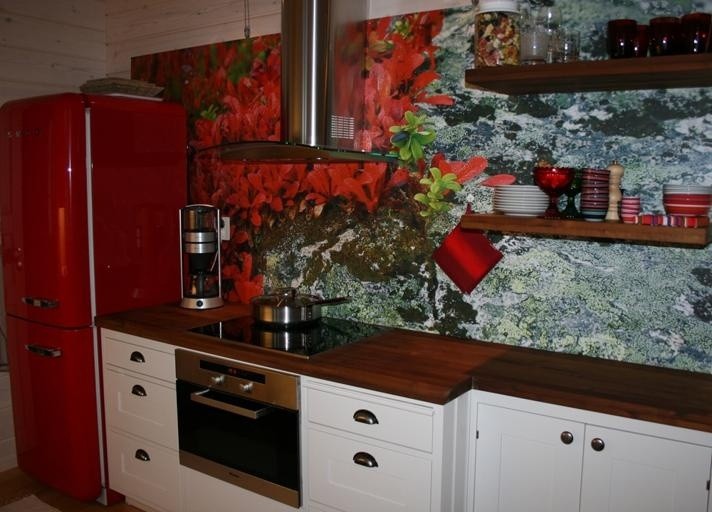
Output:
[580,168,712,224]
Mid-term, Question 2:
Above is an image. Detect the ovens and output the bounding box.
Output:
[174,346,303,509]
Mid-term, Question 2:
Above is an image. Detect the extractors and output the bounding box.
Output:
[200,1,403,168]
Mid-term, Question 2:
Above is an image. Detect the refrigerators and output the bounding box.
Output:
[0,91,189,502]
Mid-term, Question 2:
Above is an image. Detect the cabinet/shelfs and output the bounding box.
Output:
[301,378,468,512]
[471,402,712,512]
[459,51,712,246]
[100,328,179,512]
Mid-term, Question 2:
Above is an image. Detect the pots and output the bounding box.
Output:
[250,286,354,326]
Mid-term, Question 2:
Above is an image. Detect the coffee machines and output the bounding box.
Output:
[176,203,224,309]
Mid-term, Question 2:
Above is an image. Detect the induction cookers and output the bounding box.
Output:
[184,315,393,360]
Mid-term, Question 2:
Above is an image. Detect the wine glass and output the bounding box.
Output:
[536,166,586,219]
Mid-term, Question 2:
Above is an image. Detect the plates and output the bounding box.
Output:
[493,183,551,216]
[80,76,164,96]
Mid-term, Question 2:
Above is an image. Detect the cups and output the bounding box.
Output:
[474,1,712,70]
